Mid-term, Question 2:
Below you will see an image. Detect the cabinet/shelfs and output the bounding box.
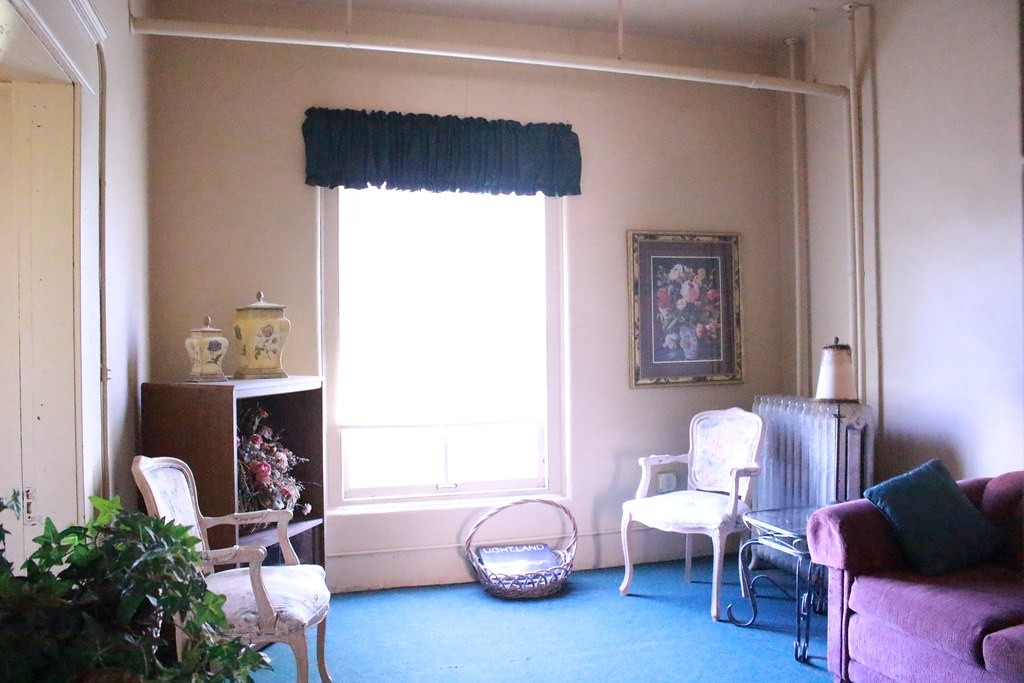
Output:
[140,375,325,571]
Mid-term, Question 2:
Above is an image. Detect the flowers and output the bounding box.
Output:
[236,403,324,534]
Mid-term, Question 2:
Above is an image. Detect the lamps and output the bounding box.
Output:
[815,336,859,508]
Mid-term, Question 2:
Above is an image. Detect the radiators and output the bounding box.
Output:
[748,394,874,612]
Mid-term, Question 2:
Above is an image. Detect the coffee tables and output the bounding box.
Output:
[727,506,830,662]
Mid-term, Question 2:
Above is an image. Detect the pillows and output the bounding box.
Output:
[864,458,1002,579]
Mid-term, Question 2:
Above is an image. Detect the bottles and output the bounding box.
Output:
[233,291,291,379]
[184,316,229,383]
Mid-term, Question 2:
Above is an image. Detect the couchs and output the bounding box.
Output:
[808,470,1024,683]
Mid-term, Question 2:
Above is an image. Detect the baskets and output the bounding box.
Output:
[464,497,578,600]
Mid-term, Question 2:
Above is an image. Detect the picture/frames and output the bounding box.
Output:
[625,229,744,387]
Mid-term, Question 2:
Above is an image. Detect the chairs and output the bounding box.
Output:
[130,454,332,683]
[619,406,763,621]
